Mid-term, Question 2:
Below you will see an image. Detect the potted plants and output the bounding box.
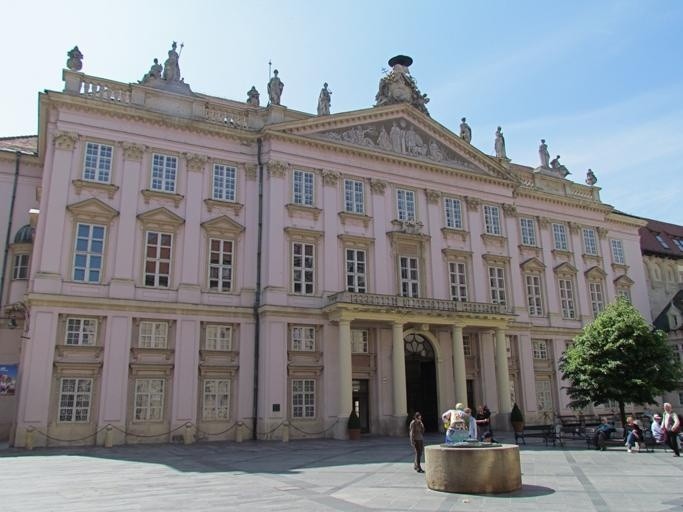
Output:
[347,409,361,441]
[510,402,524,432]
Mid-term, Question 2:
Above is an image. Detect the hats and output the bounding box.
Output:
[654,414,661,419]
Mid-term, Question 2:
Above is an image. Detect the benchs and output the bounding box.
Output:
[514,412,683,453]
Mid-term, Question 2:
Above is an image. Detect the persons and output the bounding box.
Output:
[458,118,471,145]
[164,42,180,82]
[592,416,615,452]
[317,83,332,116]
[552,411,562,432]
[441,402,471,445]
[542,411,552,426]
[659,401,682,457]
[474,405,490,434]
[246,86,260,105]
[575,410,590,439]
[550,155,560,168]
[408,411,425,473]
[538,138,550,168]
[622,415,642,452]
[480,431,499,443]
[149,58,161,79]
[266,70,284,105]
[493,126,506,158]
[584,168,597,185]
[340,121,445,164]
[651,414,665,445]
[462,407,478,441]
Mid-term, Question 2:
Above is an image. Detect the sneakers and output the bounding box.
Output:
[673,452,679,457]
[601,447,607,451]
[415,466,424,473]
[625,442,632,453]
